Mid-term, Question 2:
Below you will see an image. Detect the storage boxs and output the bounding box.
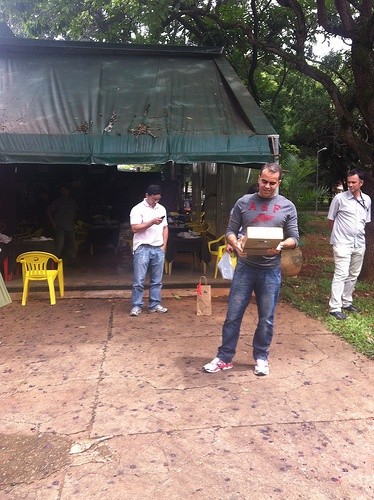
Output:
[242,227,284,256]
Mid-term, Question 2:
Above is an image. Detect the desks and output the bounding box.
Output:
[75,235,95,256]
[3,240,57,282]
[92,223,119,236]
[165,235,207,275]
[117,238,133,254]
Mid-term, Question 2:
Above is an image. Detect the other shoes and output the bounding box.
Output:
[333,312,347,320]
[346,305,360,314]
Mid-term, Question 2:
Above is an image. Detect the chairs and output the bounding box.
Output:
[207,234,237,280]
[15,251,64,306]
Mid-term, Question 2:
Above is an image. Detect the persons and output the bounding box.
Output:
[202,164,301,377]
[327,168,373,317]
[45,182,78,267]
[128,184,169,316]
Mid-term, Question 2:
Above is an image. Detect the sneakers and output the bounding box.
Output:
[253,357,268,375]
[203,357,233,373]
[150,305,167,313]
[130,307,141,317]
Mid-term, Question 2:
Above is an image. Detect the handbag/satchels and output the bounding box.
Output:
[196,276,212,315]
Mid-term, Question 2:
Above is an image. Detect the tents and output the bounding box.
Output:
[0,41,279,297]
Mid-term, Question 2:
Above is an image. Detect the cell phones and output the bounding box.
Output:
[161,216,165,219]
